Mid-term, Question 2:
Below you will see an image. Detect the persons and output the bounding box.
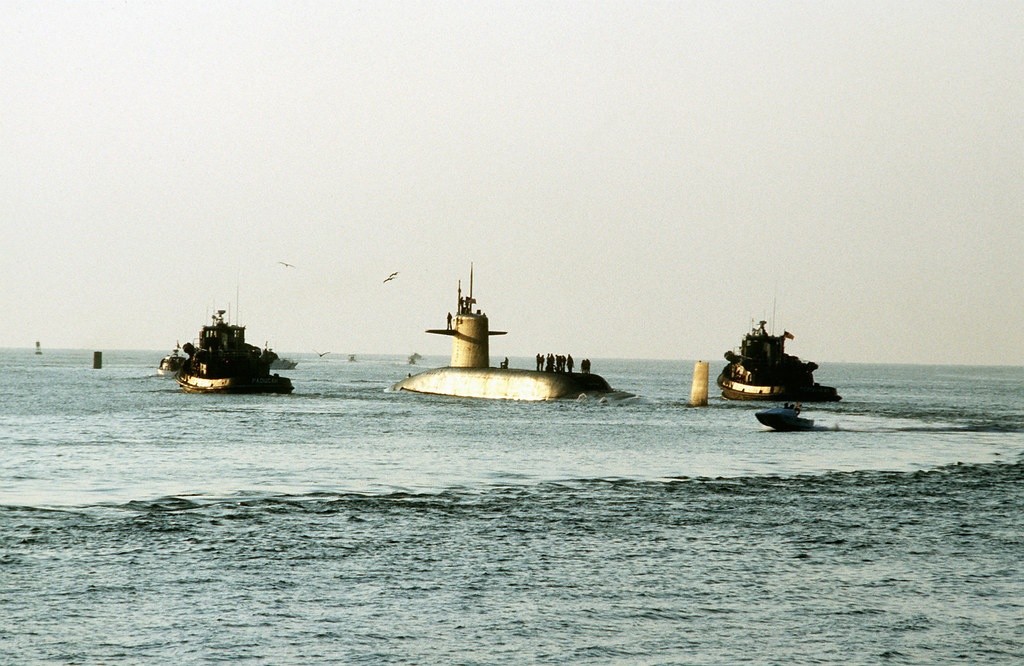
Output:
[446,311,453,331]
[536,353,591,375]
[460,297,464,309]
[783,403,794,409]
[504,357,508,369]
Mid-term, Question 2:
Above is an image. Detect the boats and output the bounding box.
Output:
[717,297,842,403]
[755,408,814,431]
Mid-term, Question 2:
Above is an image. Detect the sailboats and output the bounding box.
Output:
[156,269,297,395]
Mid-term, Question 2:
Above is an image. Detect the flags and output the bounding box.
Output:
[784,331,794,340]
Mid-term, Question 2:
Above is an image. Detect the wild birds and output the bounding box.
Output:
[383,272,399,282]
[279,261,295,268]
[313,349,331,357]
